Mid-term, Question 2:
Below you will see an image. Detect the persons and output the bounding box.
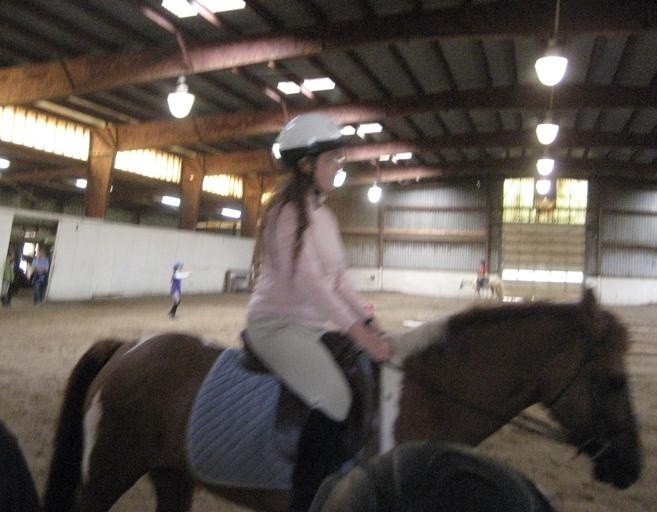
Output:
[168,262,190,318]
[0,253,15,307]
[30,247,49,306]
[245,111,391,512]
[476,259,486,289]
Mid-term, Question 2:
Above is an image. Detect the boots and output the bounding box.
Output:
[169,304,180,318]
[289,407,333,512]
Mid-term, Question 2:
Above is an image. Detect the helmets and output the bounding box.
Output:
[175,261,183,269]
[271,110,344,155]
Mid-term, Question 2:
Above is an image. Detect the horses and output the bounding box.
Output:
[41,289,639,512]
